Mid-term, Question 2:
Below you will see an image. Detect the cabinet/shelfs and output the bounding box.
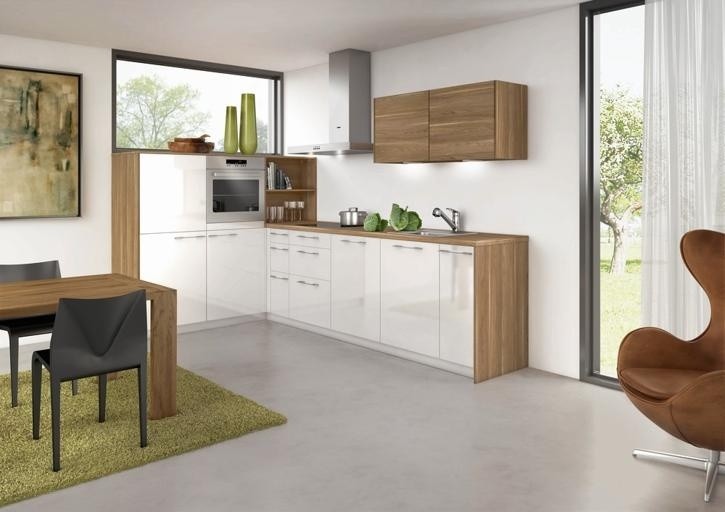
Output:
[268,154,318,225]
[110,152,206,233]
[331,233,440,369]
[372,78,530,167]
[289,224,331,339]
[267,223,289,322]
[439,236,528,385]
[111,226,267,340]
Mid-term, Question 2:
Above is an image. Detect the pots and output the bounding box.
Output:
[338,206,368,227]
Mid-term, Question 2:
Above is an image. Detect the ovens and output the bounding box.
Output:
[206,155,268,223]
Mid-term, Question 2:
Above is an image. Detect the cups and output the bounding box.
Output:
[266,161,276,190]
[275,169,284,189]
[265,200,304,223]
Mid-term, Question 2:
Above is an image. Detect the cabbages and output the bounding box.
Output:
[403,211,422,231]
[389,204,409,232]
[364,213,388,232]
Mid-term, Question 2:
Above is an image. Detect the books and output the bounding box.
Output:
[267,162,296,189]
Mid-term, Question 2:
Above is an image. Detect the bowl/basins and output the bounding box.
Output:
[166,140,213,153]
[174,137,202,143]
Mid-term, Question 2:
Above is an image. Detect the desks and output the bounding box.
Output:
[0,269,178,418]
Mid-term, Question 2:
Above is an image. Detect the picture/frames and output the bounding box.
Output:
[0,64,83,221]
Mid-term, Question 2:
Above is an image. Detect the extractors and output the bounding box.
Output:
[284,48,373,156]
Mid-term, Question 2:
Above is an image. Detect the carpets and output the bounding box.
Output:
[0,354,287,504]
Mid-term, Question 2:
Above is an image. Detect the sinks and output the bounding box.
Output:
[404,230,480,237]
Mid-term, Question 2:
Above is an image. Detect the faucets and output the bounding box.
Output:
[432,207,460,232]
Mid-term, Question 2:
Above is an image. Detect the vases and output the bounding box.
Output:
[239,92,259,156]
[223,104,240,154]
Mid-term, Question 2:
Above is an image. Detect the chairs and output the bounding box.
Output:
[615,227,724,503]
[0,260,79,408]
[30,289,149,472]
